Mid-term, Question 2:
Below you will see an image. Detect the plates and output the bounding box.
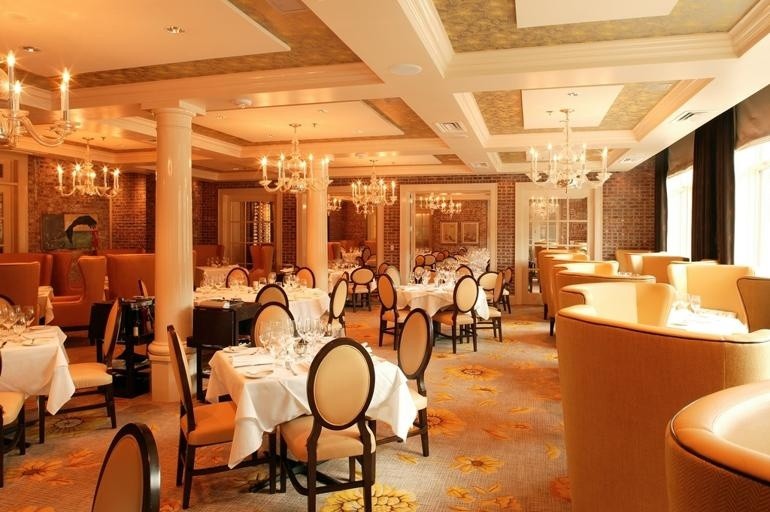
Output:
[223,341,275,379]
[23,325,52,346]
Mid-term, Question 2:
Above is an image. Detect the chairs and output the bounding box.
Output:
[326,239,376,268]
[415,247,490,272]
[92,422,161,512]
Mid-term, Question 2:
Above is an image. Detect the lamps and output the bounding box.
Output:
[530,197,559,217]
[259,123,334,193]
[419,194,461,218]
[525,104,612,195]
[356,204,375,219]
[0,51,81,150]
[327,193,342,216]
[351,159,398,205]
[55,137,123,198]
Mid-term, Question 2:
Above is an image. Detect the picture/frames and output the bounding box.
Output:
[439,220,459,245]
[461,220,480,245]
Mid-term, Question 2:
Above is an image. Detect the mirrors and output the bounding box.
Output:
[327,186,384,273]
[400,182,498,282]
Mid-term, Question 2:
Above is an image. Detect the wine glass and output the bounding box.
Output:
[198,246,491,361]
[0,303,41,345]
